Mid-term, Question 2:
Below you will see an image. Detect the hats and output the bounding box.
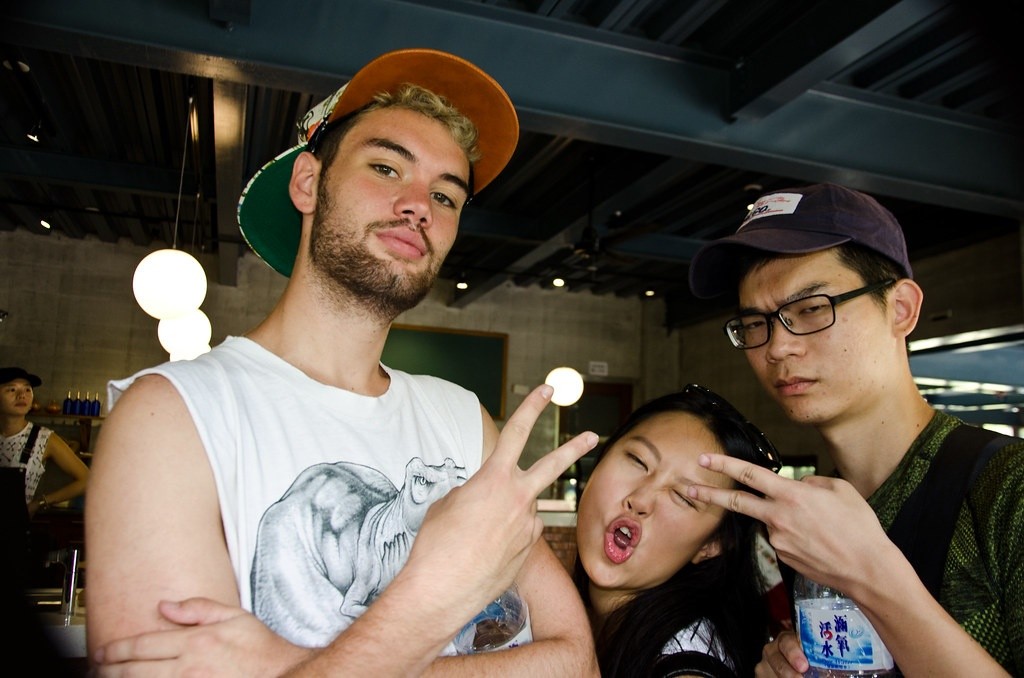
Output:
[688,182,912,298]
[0,367,43,390]
[236,49,518,278]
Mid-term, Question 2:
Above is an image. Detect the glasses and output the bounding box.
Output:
[723,277,895,350]
[684,381,782,477]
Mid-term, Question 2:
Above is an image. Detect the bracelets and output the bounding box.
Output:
[35,495,51,510]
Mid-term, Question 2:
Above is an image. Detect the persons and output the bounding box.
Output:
[571,381,782,677]
[687,181,1024,678]
[81,45,600,678]
[0,366,89,518]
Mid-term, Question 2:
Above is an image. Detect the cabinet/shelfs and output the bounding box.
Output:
[24,413,105,575]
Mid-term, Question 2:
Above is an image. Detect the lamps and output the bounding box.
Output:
[456,271,469,289]
[132,95,212,363]
[545,290,583,406]
[26,125,42,142]
[745,185,762,210]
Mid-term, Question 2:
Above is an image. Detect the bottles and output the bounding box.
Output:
[91,392,101,417]
[45,399,61,414]
[31,398,40,411]
[562,463,580,503]
[72,391,82,415]
[793,572,894,678]
[62,389,73,414]
[453,580,533,656]
[81,391,92,416]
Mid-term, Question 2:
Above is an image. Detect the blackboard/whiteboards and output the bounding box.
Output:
[380,323,509,421]
